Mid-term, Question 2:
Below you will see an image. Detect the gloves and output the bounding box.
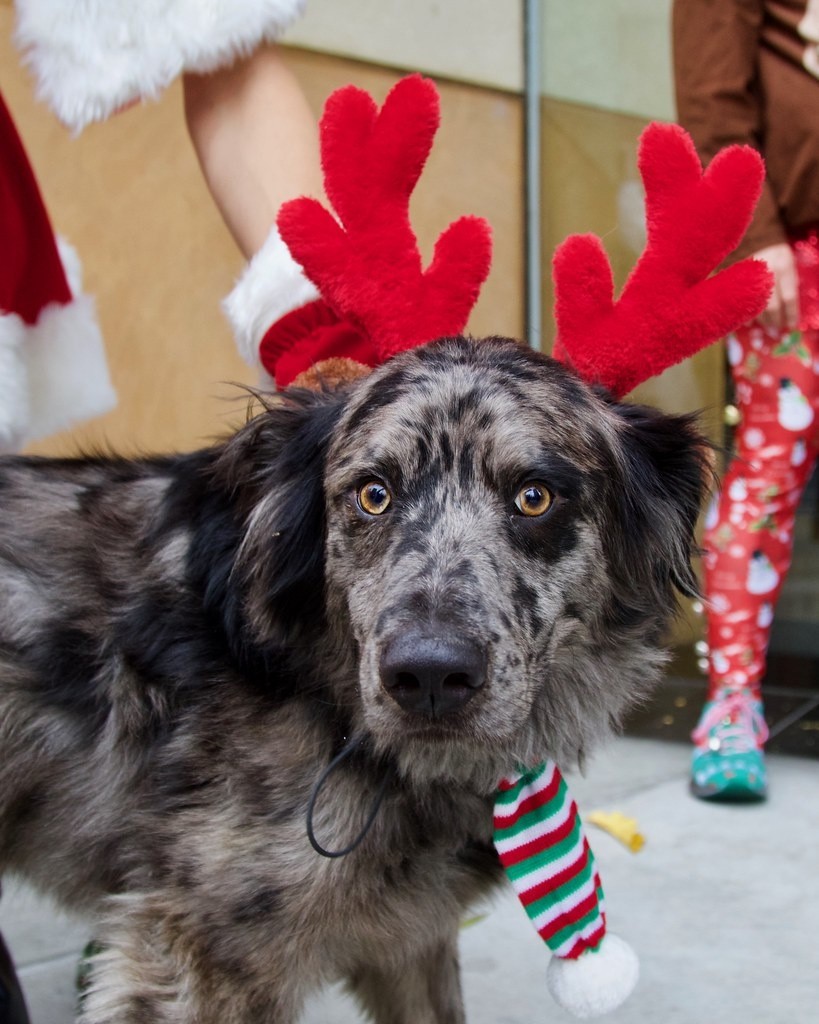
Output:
[220,209,378,385]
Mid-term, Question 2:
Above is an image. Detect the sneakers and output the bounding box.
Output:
[691,698,768,802]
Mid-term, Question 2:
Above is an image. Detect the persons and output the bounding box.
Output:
[1,1,385,456]
[671,1,819,803]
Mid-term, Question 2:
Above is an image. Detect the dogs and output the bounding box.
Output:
[0,333,724,1024]
